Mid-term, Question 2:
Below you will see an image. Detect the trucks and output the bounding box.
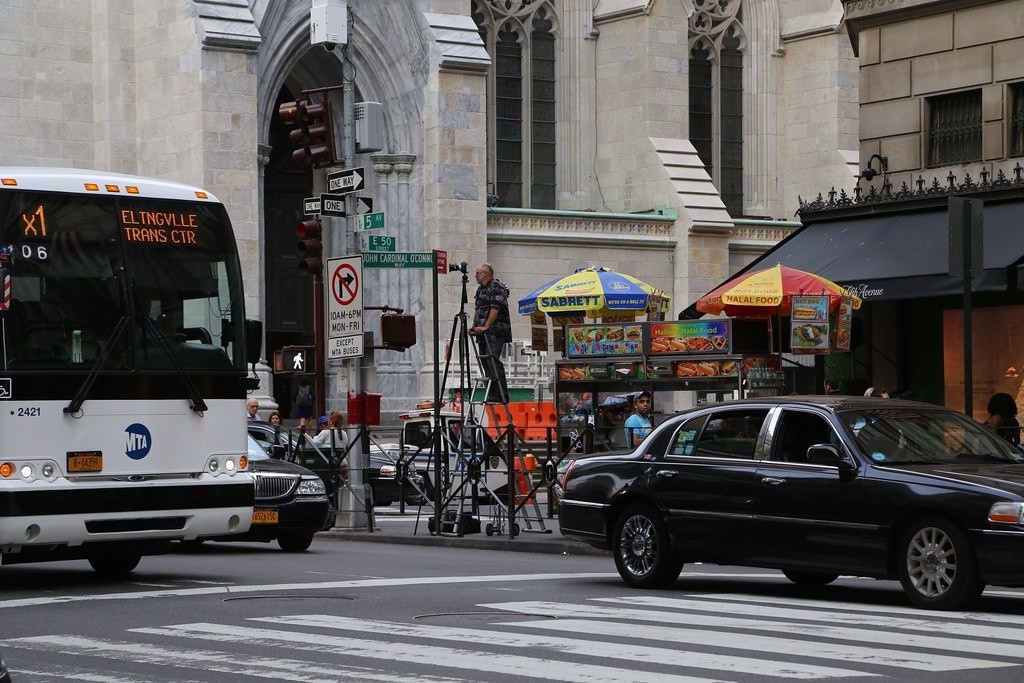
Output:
[368,407,508,505]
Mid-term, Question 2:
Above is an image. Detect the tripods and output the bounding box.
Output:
[415,273,474,536]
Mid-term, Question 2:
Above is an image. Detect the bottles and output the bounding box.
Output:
[748,367,785,388]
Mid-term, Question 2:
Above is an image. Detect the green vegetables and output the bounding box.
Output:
[793,323,827,346]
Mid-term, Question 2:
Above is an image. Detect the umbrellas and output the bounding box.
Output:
[517,264,671,323]
[696,261,863,369]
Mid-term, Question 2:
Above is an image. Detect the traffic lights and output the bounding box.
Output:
[274,349,306,374]
[279,97,311,162]
[301,101,336,163]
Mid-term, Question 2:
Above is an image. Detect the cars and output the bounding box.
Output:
[248,421,423,506]
[180,430,329,551]
[558,395,1024,612]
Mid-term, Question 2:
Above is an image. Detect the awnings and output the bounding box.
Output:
[744,203,1024,299]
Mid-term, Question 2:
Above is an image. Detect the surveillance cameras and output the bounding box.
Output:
[863,168,877,181]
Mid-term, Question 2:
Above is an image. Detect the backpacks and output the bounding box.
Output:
[298,385,310,402]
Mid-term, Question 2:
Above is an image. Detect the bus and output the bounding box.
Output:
[0,167,264,573]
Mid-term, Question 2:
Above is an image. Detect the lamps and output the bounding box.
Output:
[862,154,889,180]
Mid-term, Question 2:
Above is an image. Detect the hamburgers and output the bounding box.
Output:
[606,326,642,341]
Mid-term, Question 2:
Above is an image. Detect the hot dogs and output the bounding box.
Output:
[561,368,586,379]
[794,306,816,319]
[841,306,847,318]
[677,361,737,376]
[651,338,689,352]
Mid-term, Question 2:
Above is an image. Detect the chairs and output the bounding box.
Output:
[783,415,827,463]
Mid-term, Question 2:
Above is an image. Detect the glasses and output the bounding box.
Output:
[330,411,335,418]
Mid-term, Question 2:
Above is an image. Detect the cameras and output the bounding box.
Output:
[449,262,467,273]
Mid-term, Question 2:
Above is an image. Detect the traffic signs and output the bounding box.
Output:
[321,193,373,218]
[326,167,366,194]
[303,196,321,215]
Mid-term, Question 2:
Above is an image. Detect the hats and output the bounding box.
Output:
[633,391,651,402]
[318,416,329,425]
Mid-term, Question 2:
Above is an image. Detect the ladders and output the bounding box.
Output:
[430,328,552,537]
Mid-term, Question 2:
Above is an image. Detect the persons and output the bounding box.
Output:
[625,392,652,449]
[853,388,890,433]
[468,263,512,404]
[824,378,843,395]
[114,295,187,343]
[267,412,284,443]
[247,398,260,421]
[297,411,348,479]
[987,393,1020,445]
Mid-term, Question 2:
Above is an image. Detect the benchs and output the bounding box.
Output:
[692,438,757,455]
